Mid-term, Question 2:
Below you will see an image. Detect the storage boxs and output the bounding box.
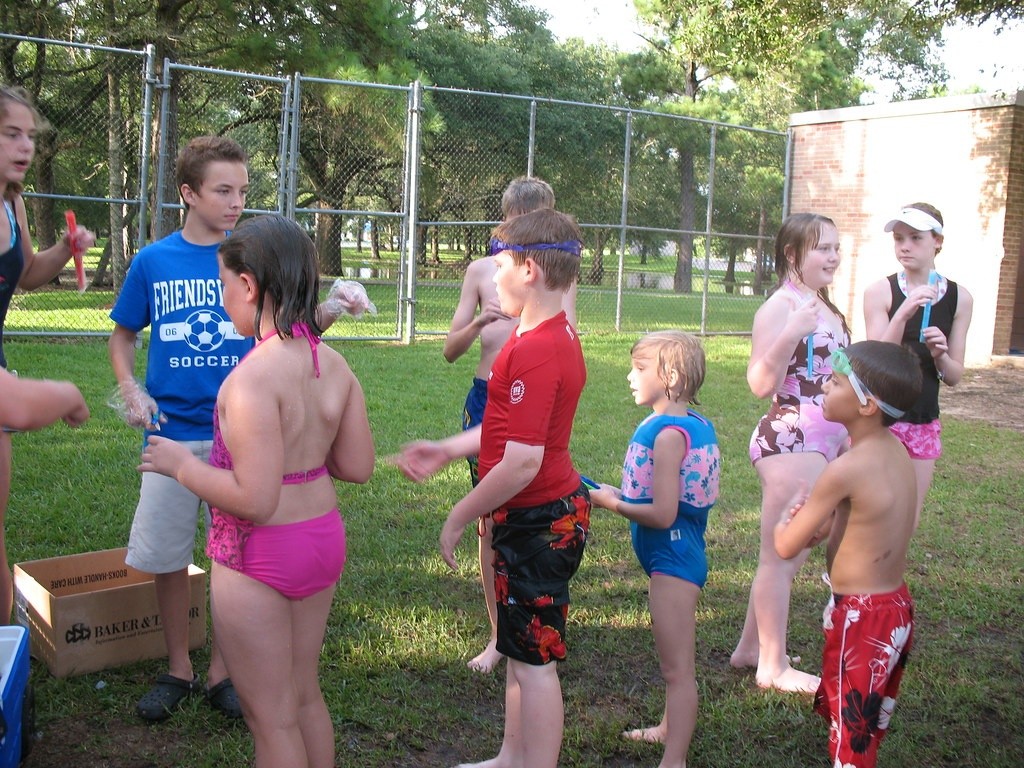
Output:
[0,624,36,768]
[14,546,206,680]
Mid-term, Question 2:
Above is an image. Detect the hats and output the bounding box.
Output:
[884,208,943,236]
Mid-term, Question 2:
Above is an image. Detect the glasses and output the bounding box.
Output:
[490,236,508,255]
[829,347,852,376]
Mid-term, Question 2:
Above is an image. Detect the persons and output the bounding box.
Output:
[443,175,577,674]
[0,368,90,431]
[728,212,851,692]
[108,137,379,723]
[822,201,973,629]
[580,330,722,768]
[134,213,376,768]
[391,210,591,767]
[774,341,922,767]
[0,82,96,626]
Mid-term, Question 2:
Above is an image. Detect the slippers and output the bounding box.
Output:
[137,673,200,720]
[204,677,243,718]
